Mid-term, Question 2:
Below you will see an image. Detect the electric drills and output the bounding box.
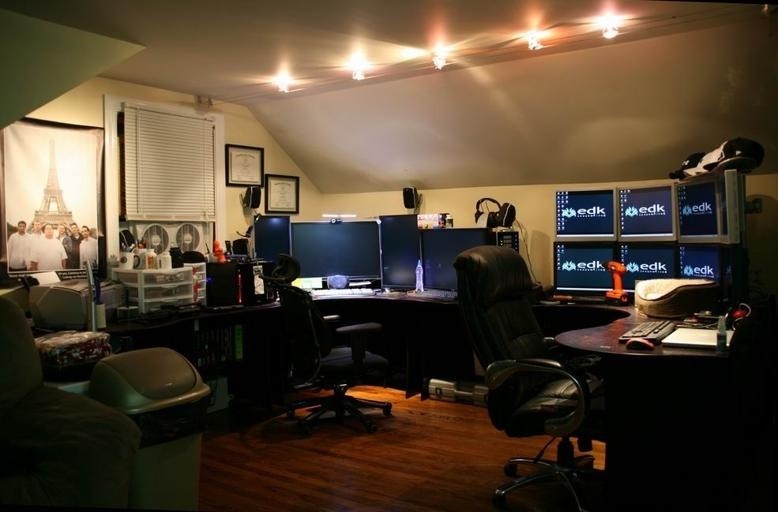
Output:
[602,261,629,305]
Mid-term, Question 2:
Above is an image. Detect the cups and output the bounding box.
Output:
[119,249,144,270]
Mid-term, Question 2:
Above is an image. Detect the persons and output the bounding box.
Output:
[7,219,98,272]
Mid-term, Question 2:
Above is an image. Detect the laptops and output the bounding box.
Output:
[660,328,735,349]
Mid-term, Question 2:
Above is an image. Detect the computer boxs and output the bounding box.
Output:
[497,231,519,254]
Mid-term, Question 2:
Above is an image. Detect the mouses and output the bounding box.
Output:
[625,338,656,352]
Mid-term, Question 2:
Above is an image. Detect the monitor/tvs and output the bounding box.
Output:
[252,214,291,263]
[290,219,382,281]
[420,228,496,291]
[550,169,746,300]
[379,214,421,289]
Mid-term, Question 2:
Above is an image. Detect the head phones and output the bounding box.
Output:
[475,197,516,228]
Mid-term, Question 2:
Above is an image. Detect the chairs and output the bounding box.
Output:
[0,294,143,511]
[451,241,606,512]
[276,283,393,433]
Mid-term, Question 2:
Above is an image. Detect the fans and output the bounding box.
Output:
[128,221,214,261]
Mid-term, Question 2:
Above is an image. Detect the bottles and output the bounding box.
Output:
[414,259,425,292]
[146,248,173,270]
[715,315,729,357]
[236,269,245,305]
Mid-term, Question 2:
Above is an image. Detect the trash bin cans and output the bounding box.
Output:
[89,346,212,512]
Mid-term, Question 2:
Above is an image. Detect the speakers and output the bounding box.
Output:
[243,186,261,209]
[403,187,419,208]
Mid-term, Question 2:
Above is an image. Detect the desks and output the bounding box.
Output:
[98,291,778,510]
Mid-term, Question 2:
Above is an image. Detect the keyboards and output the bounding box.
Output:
[311,288,375,297]
[617,320,676,343]
[407,289,458,300]
